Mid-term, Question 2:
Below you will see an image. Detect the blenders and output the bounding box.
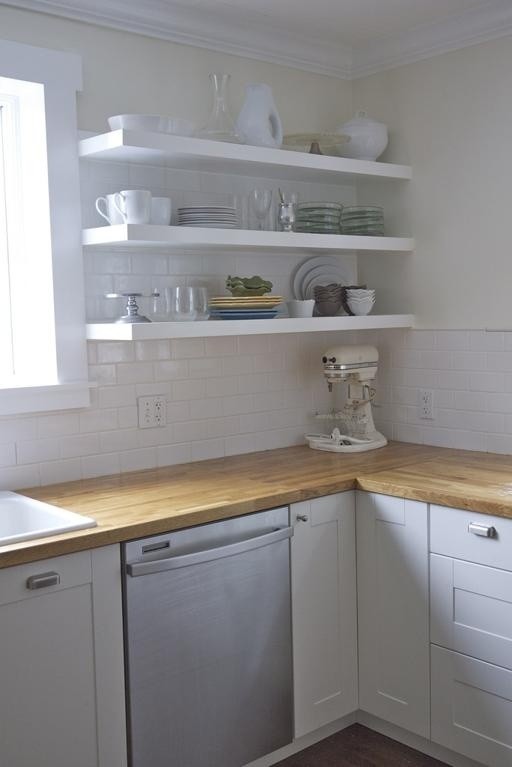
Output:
[304,341,391,454]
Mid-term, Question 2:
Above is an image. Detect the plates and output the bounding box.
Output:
[293,200,386,236]
[176,205,241,229]
[206,275,284,320]
[290,254,352,314]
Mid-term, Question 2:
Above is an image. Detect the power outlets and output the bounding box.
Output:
[419,393,434,419]
[137,394,166,429]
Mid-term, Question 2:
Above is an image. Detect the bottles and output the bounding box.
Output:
[236,81,283,149]
[193,70,248,145]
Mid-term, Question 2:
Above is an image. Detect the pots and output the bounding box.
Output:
[339,109,390,163]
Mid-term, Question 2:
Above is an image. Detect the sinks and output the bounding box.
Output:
[0,490,98,549]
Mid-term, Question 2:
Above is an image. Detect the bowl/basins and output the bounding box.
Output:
[313,284,377,316]
[107,113,179,135]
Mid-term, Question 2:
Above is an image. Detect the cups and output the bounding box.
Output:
[94,193,125,225]
[151,197,173,225]
[147,286,176,321]
[193,286,210,320]
[247,187,273,231]
[174,286,197,322]
[286,299,316,318]
[113,189,152,224]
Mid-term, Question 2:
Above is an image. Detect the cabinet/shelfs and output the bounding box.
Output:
[76,127,419,342]
[290,491,362,739]
[0,543,126,767]
[429,504,510,763]
[354,494,431,745]
